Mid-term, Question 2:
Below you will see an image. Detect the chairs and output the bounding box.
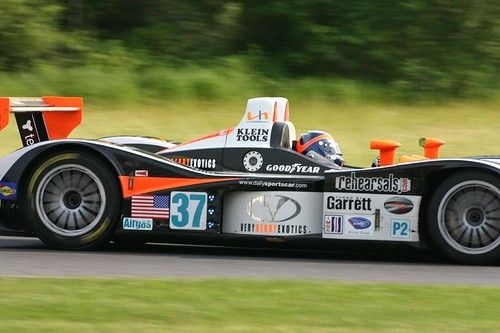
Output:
[271,122,290,148]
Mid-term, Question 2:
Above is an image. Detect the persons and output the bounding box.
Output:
[293,131,381,169]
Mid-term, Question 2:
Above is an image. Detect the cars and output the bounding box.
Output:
[0,96,500,266]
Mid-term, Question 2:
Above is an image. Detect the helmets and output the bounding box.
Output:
[296,130,345,167]
